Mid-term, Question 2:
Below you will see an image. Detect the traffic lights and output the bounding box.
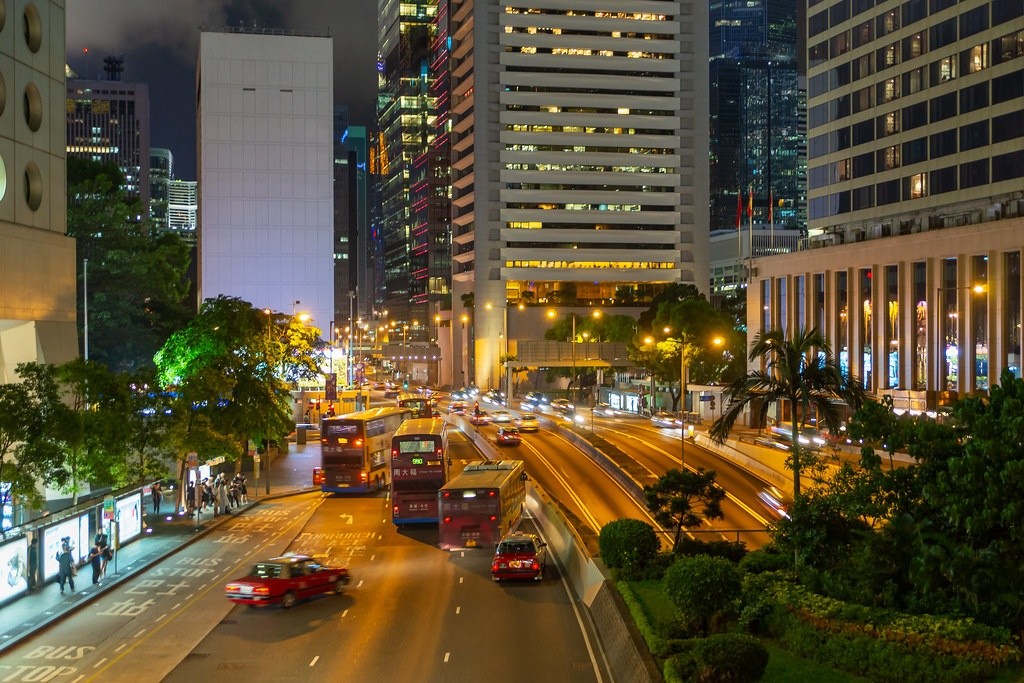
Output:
[315,399,320,409]
[402,380,409,389]
[474,403,479,415]
[328,405,335,416]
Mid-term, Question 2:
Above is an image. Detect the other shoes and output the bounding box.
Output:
[72,589,75,592]
[186,502,249,517]
[60,590,63,593]
[28,588,35,591]
[94,582,100,585]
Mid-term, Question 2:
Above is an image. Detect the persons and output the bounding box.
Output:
[186,470,249,517]
[59,546,77,592]
[151,483,163,516]
[90,526,112,584]
[304,412,309,424]
[28,538,38,588]
[771,418,779,428]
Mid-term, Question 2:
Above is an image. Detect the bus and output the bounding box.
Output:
[322,407,412,493]
[396,393,431,427]
[437,459,526,552]
[391,418,452,526]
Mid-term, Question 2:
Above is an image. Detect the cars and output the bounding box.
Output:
[497,426,521,446]
[514,413,540,432]
[591,401,621,419]
[491,531,547,583]
[469,410,512,425]
[446,401,468,415]
[651,411,680,428]
[224,551,351,609]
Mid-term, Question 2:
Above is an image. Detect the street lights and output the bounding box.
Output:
[485,302,525,406]
[644,326,726,427]
[543,308,602,410]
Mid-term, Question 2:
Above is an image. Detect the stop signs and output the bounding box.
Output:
[307,402,315,409]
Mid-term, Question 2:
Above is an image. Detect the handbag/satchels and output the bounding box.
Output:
[55,572,66,584]
[107,552,113,561]
[71,563,77,576]
